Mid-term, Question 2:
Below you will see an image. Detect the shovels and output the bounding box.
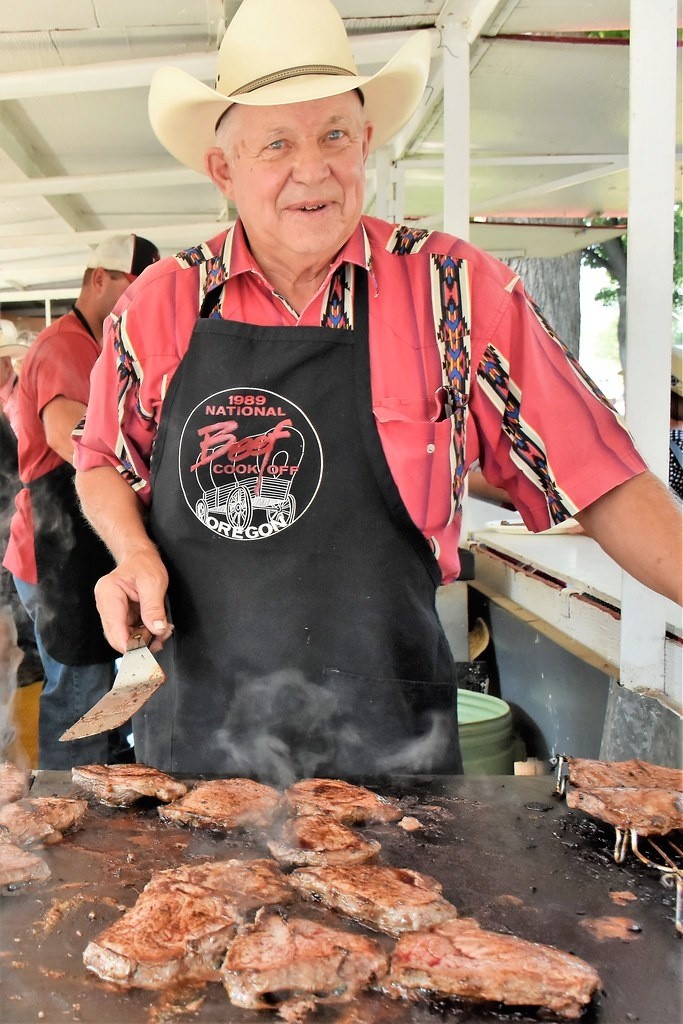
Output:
[59,619,166,742]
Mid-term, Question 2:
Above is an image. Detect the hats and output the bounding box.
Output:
[147,0,433,177]
[0,318,31,359]
[85,231,161,285]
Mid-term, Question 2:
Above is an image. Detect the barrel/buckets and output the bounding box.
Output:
[457,687,517,776]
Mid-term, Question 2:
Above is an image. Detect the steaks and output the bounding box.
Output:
[0,759,606,1024]
[564,756,683,837]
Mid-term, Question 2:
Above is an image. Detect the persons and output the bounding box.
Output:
[669,374,683,499]
[2,234,161,770]
[69,0,683,775]
[0,318,45,688]
